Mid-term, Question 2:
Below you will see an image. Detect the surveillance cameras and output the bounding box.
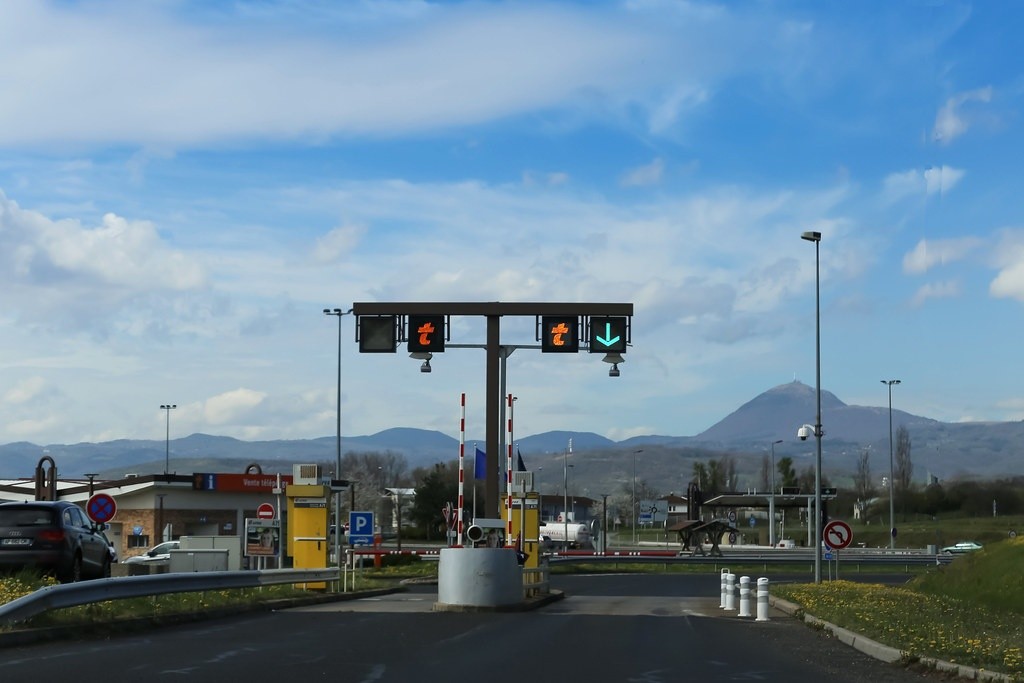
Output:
[798,427,809,441]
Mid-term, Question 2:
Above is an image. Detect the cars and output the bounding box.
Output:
[941,541,984,555]
[0,500,113,583]
[122,539,182,565]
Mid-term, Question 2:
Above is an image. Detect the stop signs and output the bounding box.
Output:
[257,504,275,522]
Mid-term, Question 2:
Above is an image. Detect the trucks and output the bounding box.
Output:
[539,522,591,549]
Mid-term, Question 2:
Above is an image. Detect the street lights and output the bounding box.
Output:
[632,450,645,544]
[801,231,823,584]
[567,465,576,521]
[323,307,355,566]
[771,439,784,547]
[881,379,901,550]
[160,403,177,474]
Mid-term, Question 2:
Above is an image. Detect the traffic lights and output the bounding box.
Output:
[589,316,628,353]
